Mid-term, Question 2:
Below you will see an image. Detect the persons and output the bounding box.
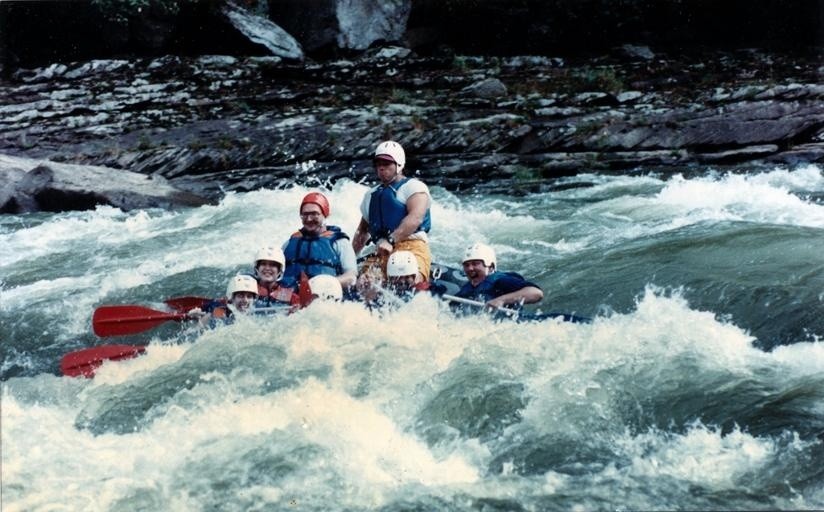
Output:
[448,244,543,315]
[356,250,447,308]
[187,246,299,319]
[351,139,432,284]
[181,276,278,333]
[296,270,344,314]
[280,193,358,300]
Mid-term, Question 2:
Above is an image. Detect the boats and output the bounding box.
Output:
[209,257,486,328]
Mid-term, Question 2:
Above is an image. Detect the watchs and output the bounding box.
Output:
[384,236,395,249]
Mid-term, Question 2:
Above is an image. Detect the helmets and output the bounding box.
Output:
[385,249,421,277]
[226,273,260,302]
[252,244,286,282]
[461,242,499,273]
[374,139,407,175]
[300,191,330,218]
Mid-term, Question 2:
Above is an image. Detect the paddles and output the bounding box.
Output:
[442,293,592,328]
[61,328,204,379]
[93,305,305,337]
[166,295,225,310]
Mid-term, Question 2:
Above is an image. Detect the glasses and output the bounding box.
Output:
[300,211,323,218]
